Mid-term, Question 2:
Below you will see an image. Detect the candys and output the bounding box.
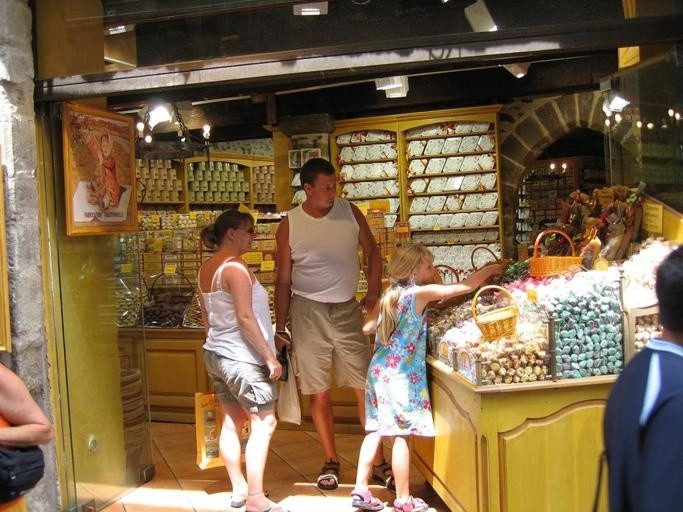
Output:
[428,262,663,384]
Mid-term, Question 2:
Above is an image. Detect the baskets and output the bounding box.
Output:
[471,247,509,270]
[528,230,584,277]
[427,265,463,308]
[472,285,518,342]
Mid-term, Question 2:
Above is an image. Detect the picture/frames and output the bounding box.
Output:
[0,165,13,355]
[59,105,140,238]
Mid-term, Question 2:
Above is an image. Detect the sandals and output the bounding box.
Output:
[351,463,428,512]
[231,490,289,512]
[317,462,340,489]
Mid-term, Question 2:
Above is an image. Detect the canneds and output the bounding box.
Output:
[135,158,275,203]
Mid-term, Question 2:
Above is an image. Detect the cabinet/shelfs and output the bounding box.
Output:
[516,156,582,246]
[113,103,511,512]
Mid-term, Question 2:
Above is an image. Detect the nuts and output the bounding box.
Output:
[115,290,189,328]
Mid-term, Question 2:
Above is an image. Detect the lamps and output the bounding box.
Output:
[528,160,567,184]
[601,90,631,117]
[372,76,413,100]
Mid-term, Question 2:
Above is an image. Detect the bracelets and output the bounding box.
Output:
[367,293,381,297]
[277,332,286,335]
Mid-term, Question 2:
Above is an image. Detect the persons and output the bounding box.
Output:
[196,210,290,512]
[86,127,120,210]
[274,158,397,491]
[0,365,52,512]
[350,244,505,512]
[602,245,683,512]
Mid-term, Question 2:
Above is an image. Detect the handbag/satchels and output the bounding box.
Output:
[276,348,288,381]
[0,446,44,502]
[194,391,250,470]
